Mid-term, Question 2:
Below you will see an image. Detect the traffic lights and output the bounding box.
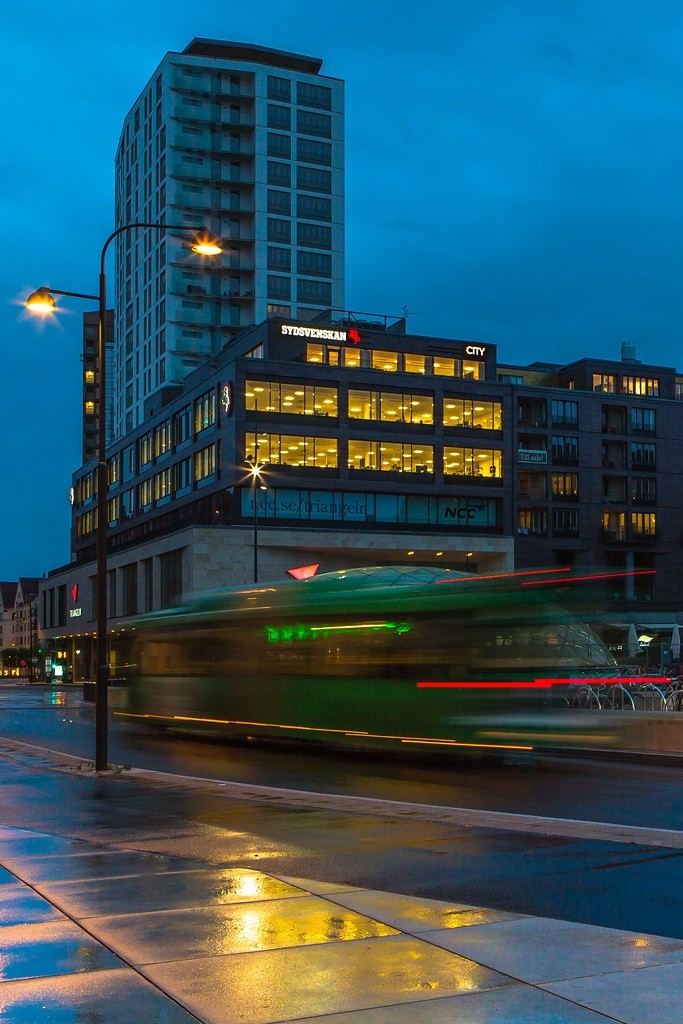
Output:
[37,647,42,653]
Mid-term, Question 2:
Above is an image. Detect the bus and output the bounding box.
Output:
[116,564,625,769]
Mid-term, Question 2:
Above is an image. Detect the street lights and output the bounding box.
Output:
[25,224,222,768]
